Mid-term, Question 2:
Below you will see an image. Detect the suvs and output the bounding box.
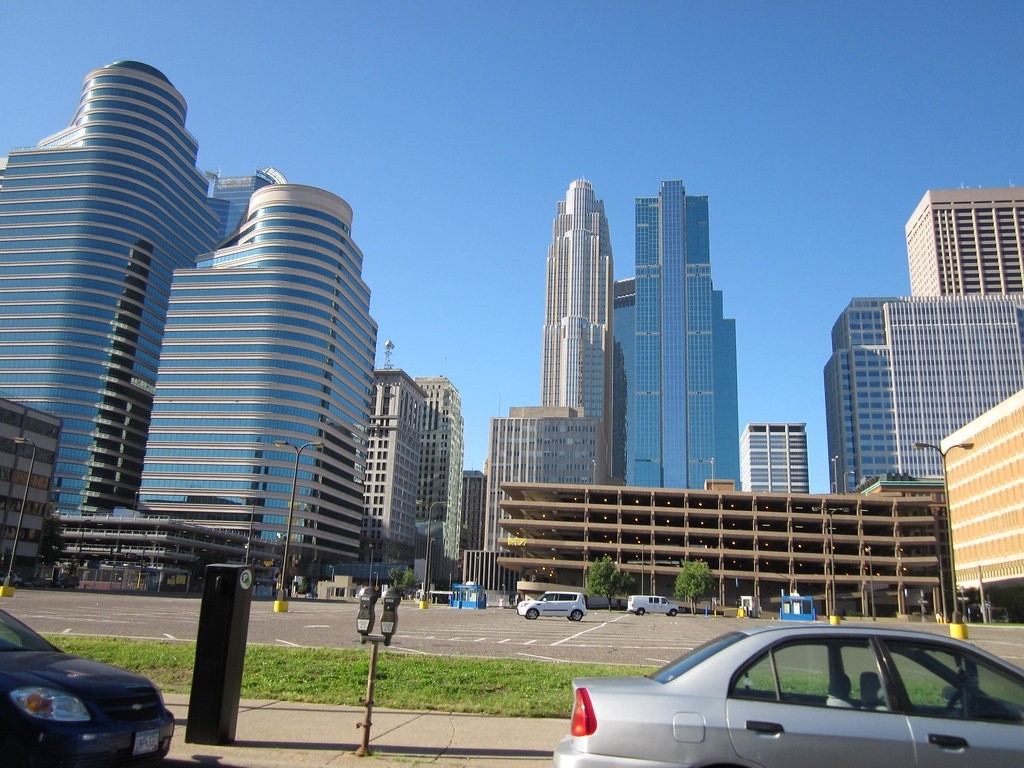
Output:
[516,589,588,622]
[628,593,679,617]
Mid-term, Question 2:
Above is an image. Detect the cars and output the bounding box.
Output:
[1,605,177,768]
[553,623,1024,768]
[0,572,82,590]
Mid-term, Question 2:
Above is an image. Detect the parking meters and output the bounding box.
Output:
[351,586,406,755]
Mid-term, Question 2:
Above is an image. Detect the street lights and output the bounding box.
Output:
[831,456,855,495]
[911,440,975,626]
[274,440,324,600]
[812,506,851,616]
[4,436,36,585]
[865,544,878,621]
[710,457,715,490]
[592,458,598,485]
[416,500,448,600]
